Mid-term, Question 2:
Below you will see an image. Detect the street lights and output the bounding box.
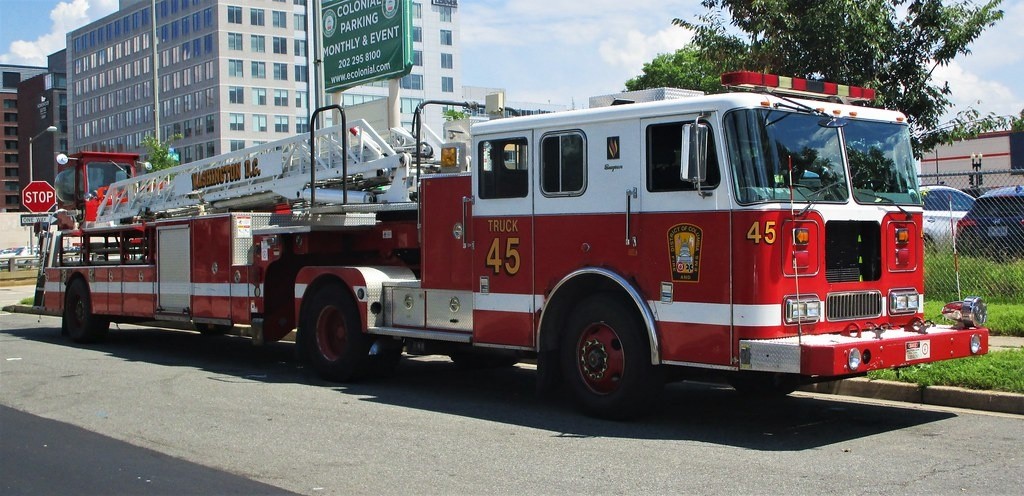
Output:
[29,125,57,254]
[971,152,982,188]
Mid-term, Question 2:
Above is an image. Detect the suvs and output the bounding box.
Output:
[955,184,1024,264]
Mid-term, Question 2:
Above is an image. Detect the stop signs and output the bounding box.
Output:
[21,180,56,213]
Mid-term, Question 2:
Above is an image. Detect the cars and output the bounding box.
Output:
[0,246,41,270]
[919,185,977,246]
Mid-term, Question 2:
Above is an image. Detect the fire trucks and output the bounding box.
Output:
[33,72,988,424]
